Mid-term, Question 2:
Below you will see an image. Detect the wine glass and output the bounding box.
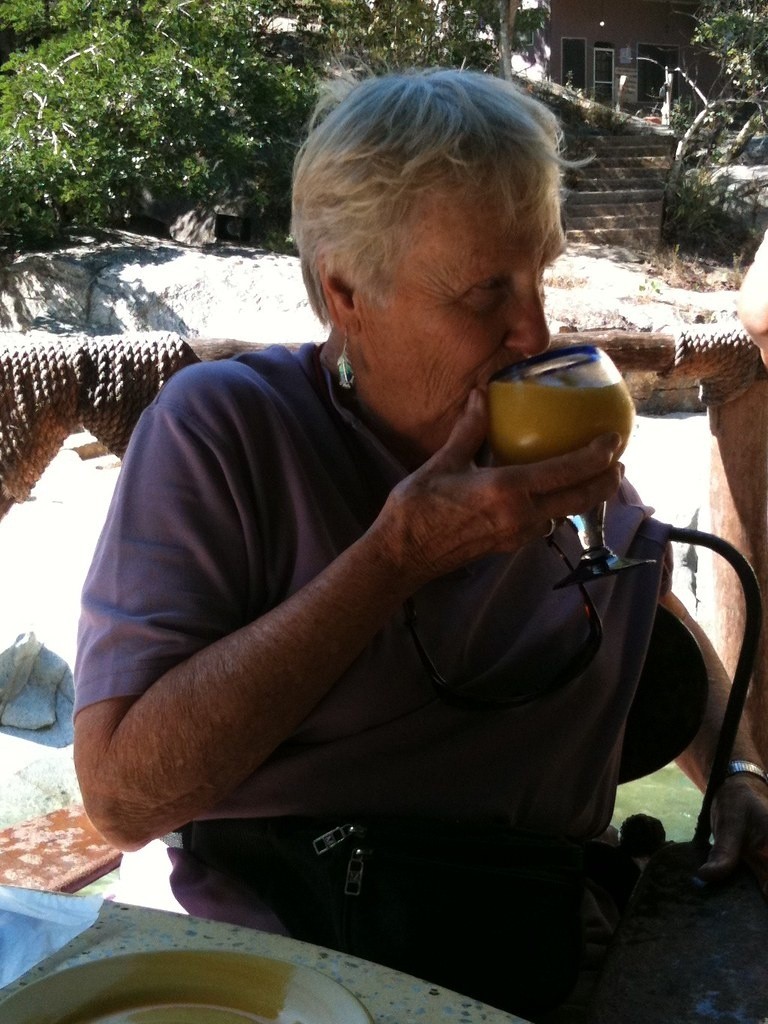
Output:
[489,343,660,589]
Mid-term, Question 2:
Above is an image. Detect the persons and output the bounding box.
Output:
[70,69,767,1023]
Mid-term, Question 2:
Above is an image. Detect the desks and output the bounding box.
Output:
[2,881,530,1023]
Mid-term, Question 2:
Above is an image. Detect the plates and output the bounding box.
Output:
[0,949,377,1024]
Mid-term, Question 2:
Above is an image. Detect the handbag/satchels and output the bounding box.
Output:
[181,812,647,1024]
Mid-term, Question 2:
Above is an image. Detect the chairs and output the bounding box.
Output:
[607,518,765,902]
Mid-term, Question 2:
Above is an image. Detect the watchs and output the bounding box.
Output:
[717,757,768,784]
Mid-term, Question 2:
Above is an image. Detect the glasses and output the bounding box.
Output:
[400,536,604,712]
